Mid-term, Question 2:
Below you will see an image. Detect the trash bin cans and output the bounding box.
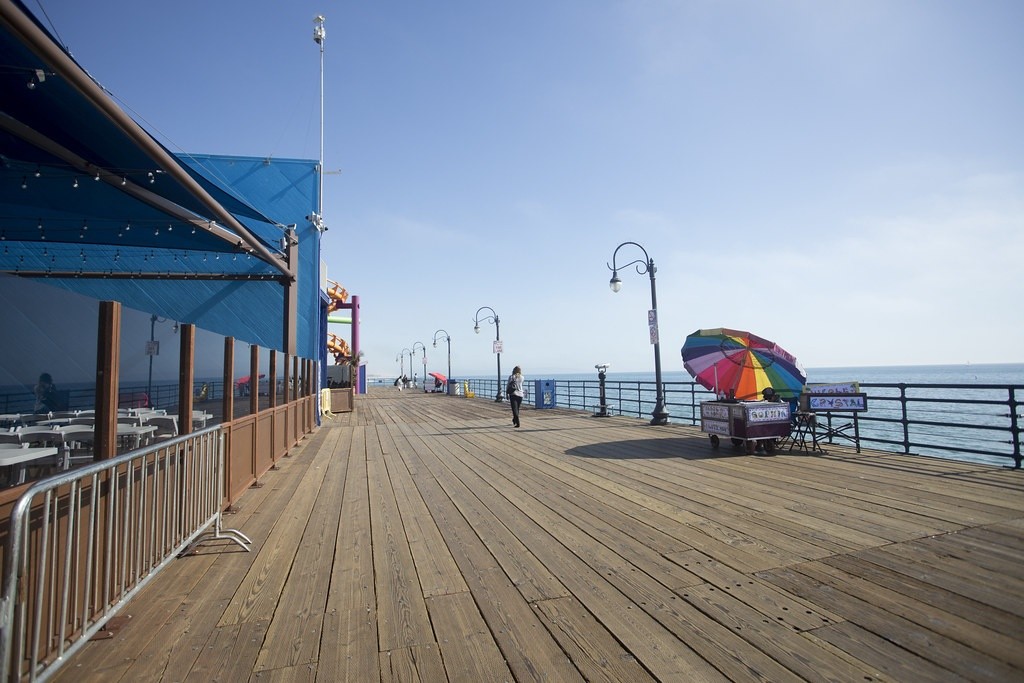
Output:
[407,381,412,388]
[535,379,556,411]
[450,382,460,395]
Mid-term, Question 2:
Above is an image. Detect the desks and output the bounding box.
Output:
[168,414,214,421]
[0,447,58,467]
[116,426,157,449]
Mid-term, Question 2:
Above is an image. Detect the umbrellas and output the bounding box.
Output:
[235,373,265,387]
[428,373,446,384]
[680,327,808,402]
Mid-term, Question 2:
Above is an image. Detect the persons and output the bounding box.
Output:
[754,387,785,449]
[34,372,56,414]
[506,366,524,427]
[435,380,440,391]
[414,373,418,389]
[396,374,407,391]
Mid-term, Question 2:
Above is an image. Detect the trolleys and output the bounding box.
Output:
[700,399,793,456]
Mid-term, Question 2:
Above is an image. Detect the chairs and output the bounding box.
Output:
[0,407,212,485]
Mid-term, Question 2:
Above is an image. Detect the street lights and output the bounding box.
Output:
[412,342,428,393]
[401,348,412,388]
[312,14,326,420]
[147,311,180,407]
[396,353,403,378]
[473,307,504,402]
[605,242,670,427]
[432,330,450,395]
